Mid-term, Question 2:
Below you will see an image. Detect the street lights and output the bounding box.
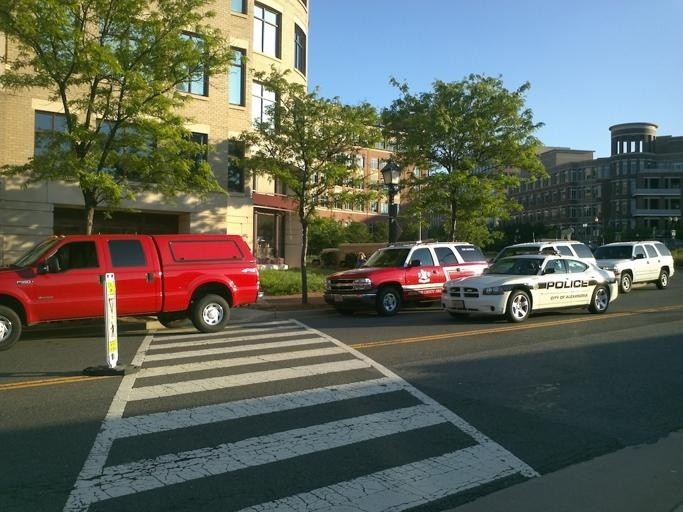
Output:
[380,156,404,246]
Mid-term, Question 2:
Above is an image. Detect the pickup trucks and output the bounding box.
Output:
[0,233,261,352]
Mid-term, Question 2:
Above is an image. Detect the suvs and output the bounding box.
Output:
[592,240,675,294]
[491,239,597,274]
[323,240,489,317]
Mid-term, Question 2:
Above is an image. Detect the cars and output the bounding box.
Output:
[439,253,619,322]
